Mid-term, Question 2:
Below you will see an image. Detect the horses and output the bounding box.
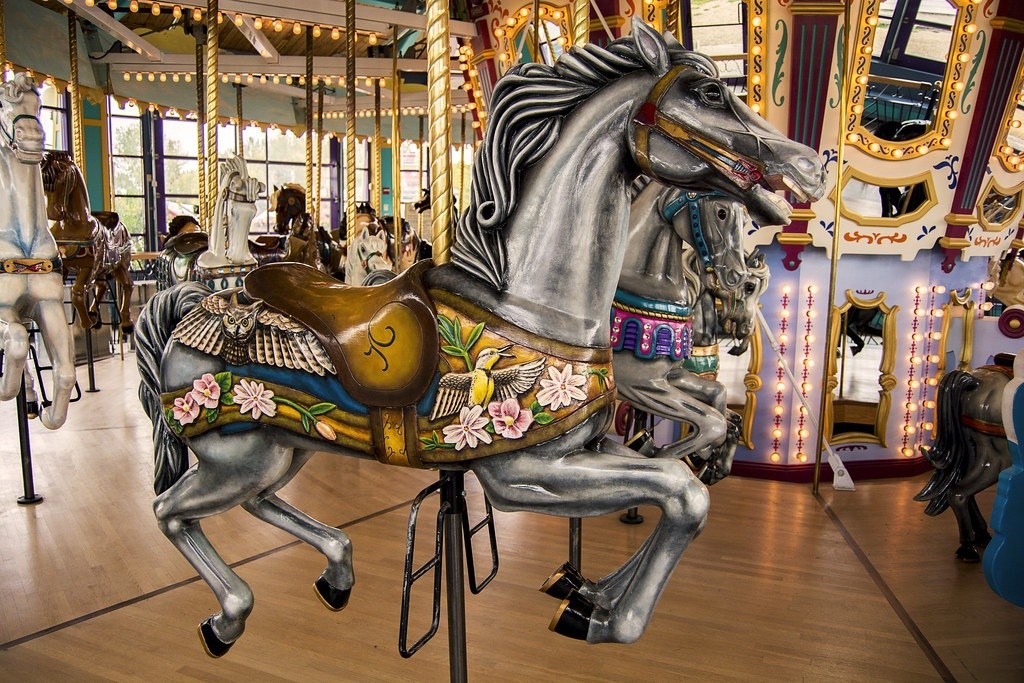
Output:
[911,351,1016,569]
[130,13,827,658]
[1,68,136,430]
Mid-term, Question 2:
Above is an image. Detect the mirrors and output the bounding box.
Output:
[493,0,1024,452]
